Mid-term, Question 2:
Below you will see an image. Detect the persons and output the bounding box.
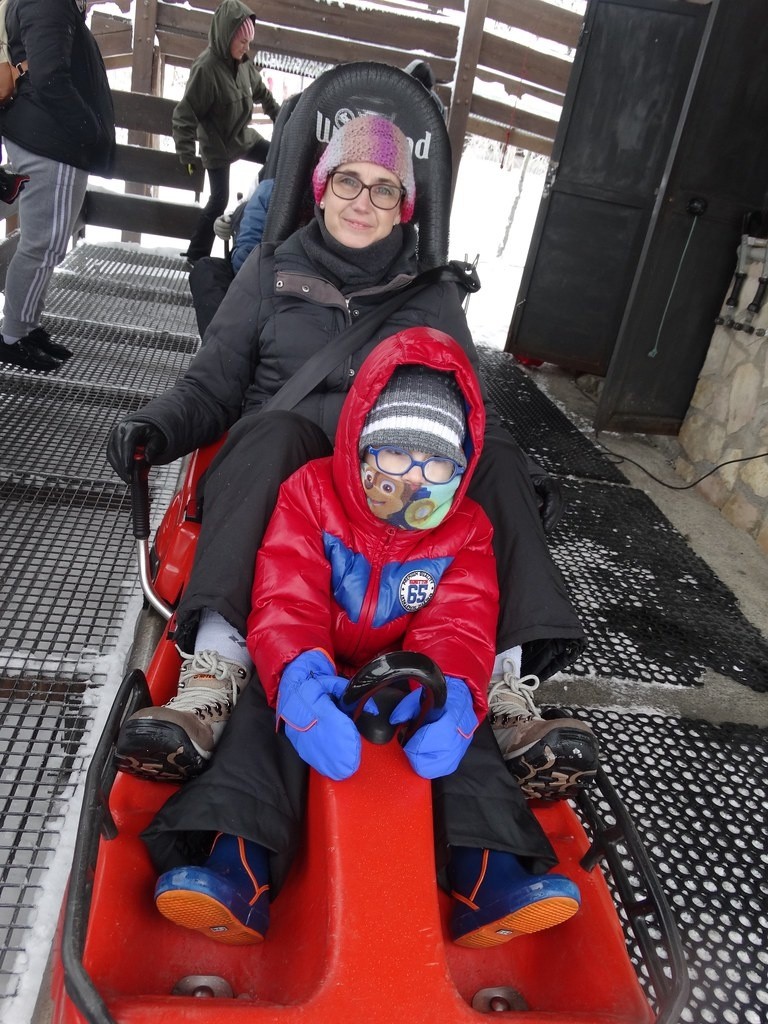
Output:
[105,116,599,947]
[171,0,281,271]
[0,0,116,371]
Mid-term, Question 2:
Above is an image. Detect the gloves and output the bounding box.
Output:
[213,215,232,241]
[389,673,479,781]
[178,164,197,176]
[105,418,169,486]
[530,475,564,535]
[273,647,378,782]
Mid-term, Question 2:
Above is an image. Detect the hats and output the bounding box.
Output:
[358,365,469,475]
[230,16,255,44]
[311,115,416,223]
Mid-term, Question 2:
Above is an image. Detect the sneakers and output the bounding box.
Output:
[485,658,600,806]
[111,644,251,786]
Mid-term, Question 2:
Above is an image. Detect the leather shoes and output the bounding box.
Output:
[29,326,74,360]
[0,335,64,367]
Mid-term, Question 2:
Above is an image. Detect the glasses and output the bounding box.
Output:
[329,170,407,211]
[365,443,466,485]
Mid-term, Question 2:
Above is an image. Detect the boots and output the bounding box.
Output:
[153,832,274,945]
[438,846,580,950]
